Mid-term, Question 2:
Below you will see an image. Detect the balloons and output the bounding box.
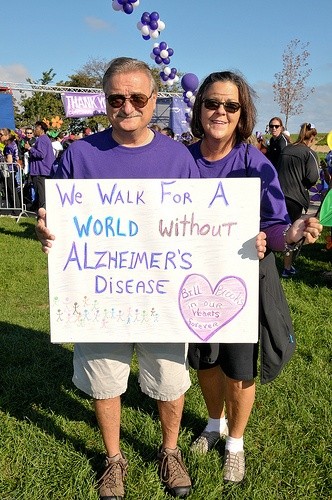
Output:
[150,41,174,65]
[160,67,179,87]
[112,0,139,14]
[137,12,166,40]
[180,72,202,121]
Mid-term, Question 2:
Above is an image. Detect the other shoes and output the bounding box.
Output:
[281,265,297,276]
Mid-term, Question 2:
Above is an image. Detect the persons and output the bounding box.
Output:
[274,124,319,279]
[267,117,291,162]
[35,57,267,500]
[175,71,323,484]
[0,120,195,220]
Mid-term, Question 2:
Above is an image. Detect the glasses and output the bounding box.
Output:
[269,125,281,128]
[105,90,155,108]
[202,98,242,114]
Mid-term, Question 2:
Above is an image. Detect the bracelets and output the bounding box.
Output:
[283,222,305,256]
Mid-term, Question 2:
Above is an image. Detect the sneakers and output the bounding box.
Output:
[191,419,228,453]
[93,451,128,500]
[221,448,245,485]
[158,443,192,499]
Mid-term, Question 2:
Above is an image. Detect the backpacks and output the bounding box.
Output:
[19,180,38,212]
